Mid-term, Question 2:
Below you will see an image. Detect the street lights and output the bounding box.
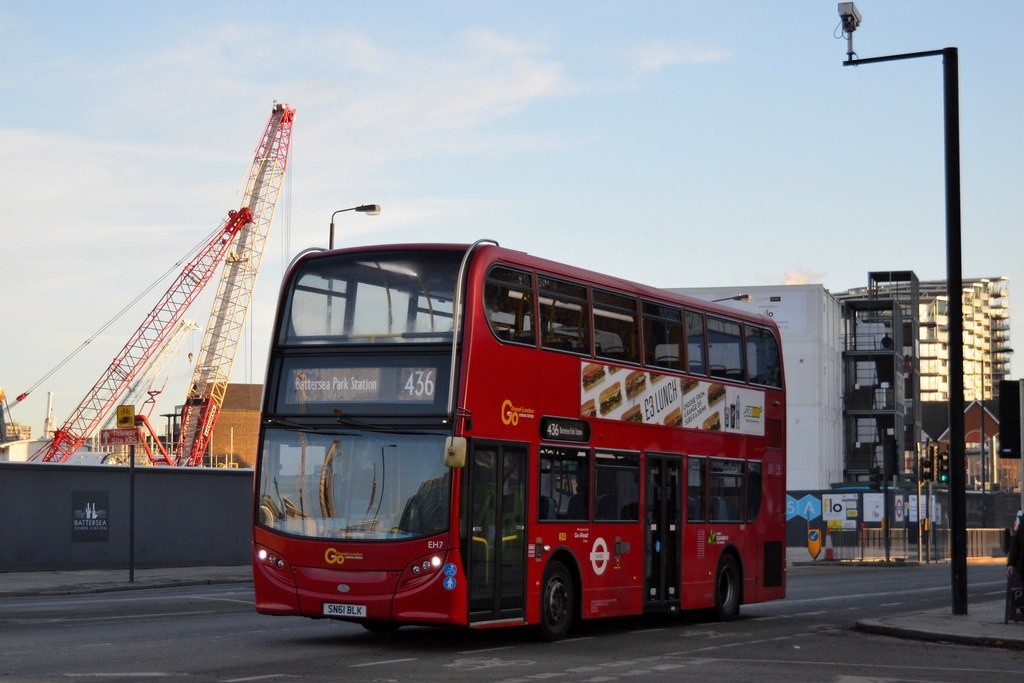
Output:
[326,203,381,334]
[980,348,1013,528]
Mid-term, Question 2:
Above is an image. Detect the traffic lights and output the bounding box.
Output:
[869,467,880,490]
[922,445,937,481]
[941,446,950,483]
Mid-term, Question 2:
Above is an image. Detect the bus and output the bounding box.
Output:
[251,238,787,645]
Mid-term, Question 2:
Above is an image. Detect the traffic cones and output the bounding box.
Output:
[820,529,839,561]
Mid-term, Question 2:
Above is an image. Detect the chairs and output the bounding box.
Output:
[492,321,768,384]
[535,486,743,518]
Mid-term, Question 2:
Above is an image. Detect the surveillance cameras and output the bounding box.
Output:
[838,2,862,27]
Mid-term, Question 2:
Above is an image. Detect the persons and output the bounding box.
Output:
[880,332,893,350]
